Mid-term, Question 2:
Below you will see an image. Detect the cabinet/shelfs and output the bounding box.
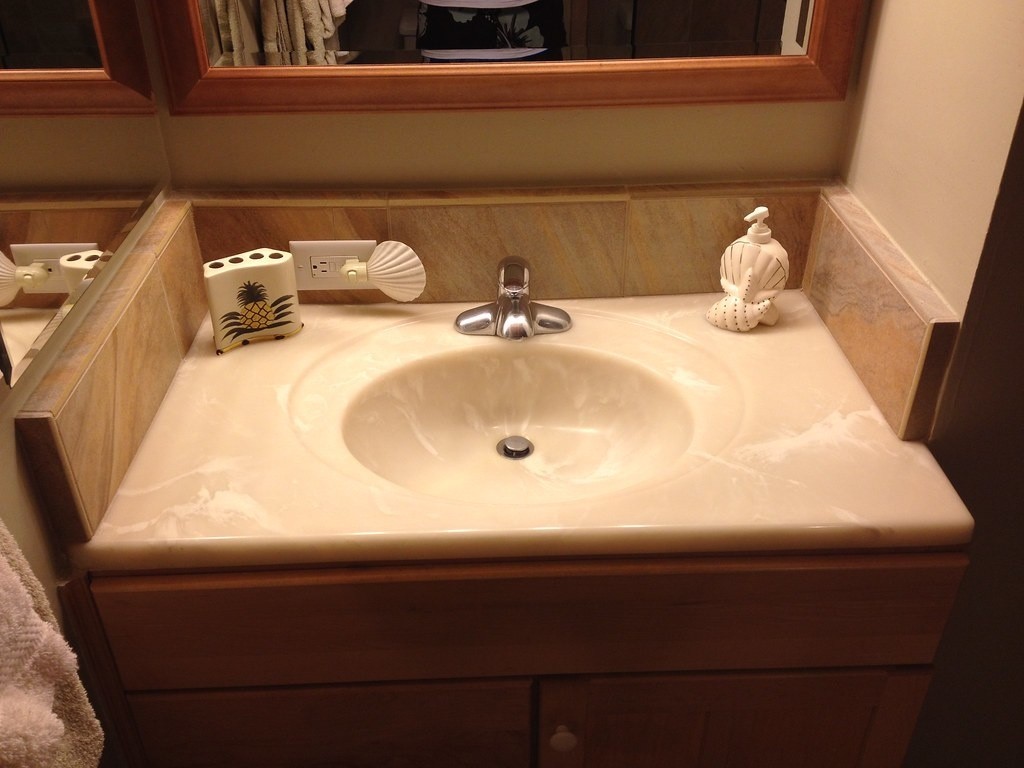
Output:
[83,548,973,768]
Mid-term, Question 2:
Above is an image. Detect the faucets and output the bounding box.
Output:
[453,255,573,342]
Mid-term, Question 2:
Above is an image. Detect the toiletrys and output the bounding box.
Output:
[704,206,791,332]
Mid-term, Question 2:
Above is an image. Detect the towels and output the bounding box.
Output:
[199,0,353,67]
[0,515,105,767]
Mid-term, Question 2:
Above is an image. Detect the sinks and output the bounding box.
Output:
[286,302,747,506]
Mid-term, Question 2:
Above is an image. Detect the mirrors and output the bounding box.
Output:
[147,0,872,117]
[0,1,174,122]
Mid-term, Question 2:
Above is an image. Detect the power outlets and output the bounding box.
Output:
[289,241,378,290]
[10,243,101,294]
